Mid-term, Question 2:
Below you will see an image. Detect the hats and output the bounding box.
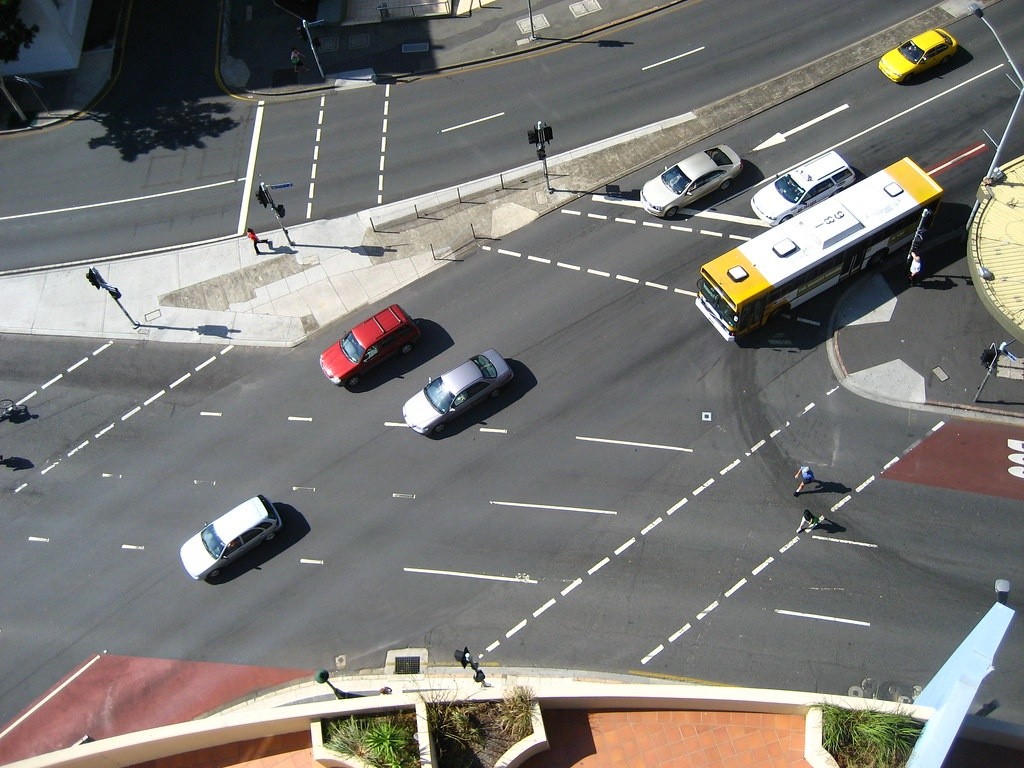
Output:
[803,467,809,473]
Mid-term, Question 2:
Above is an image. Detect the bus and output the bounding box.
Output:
[697,156,945,343]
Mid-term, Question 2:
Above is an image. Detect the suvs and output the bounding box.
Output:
[320,303,422,391]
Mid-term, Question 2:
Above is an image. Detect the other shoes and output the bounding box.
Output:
[796,527,802,533]
[809,523,814,527]
[793,491,800,497]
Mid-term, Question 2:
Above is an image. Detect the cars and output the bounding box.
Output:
[402,348,514,436]
[179,494,280,581]
[879,28,957,86]
[750,150,857,227]
[640,144,743,219]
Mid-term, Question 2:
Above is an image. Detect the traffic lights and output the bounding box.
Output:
[108,287,122,301]
[85,268,100,290]
[980,342,998,368]
[254,185,268,209]
[527,126,539,147]
[277,205,286,218]
[453,645,468,669]
[543,123,554,145]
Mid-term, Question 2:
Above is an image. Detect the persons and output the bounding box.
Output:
[246,228,272,255]
[908,252,922,287]
[796,509,817,533]
[290,47,308,72]
[792,467,823,497]
